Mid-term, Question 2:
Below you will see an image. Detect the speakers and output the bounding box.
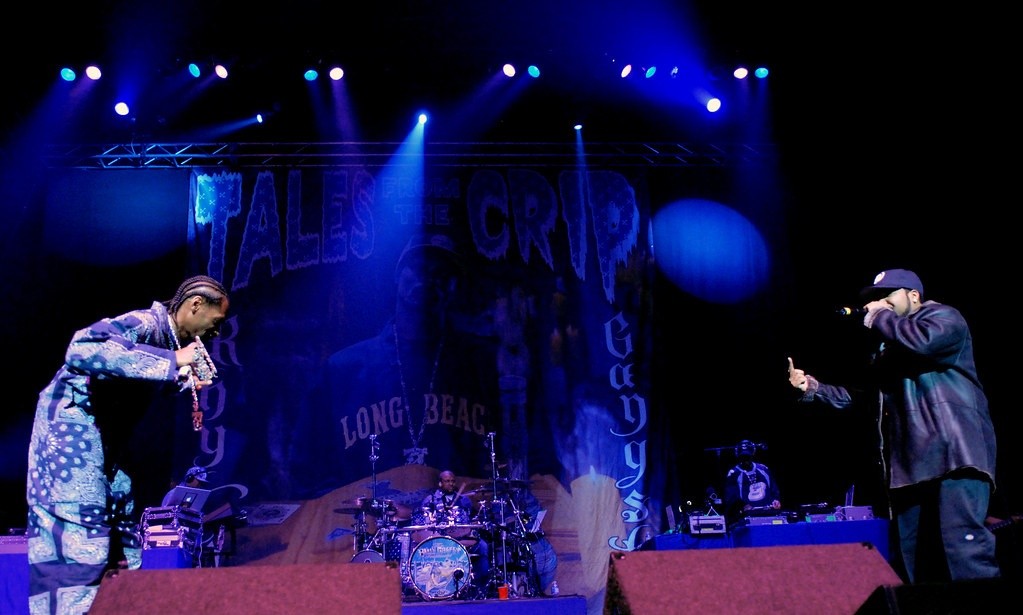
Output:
[602,541,903,615]
[87,560,403,615]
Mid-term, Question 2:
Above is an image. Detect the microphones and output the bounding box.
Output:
[836,307,869,316]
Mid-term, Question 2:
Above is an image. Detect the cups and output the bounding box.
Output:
[498,586,508,600]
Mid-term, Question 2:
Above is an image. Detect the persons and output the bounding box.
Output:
[25,276,230,615]
[418,470,472,523]
[725,440,780,518]
[787,269,998,584]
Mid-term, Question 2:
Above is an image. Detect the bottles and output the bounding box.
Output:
[551,581,560,597]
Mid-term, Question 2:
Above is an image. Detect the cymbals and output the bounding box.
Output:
[472,480,530,492]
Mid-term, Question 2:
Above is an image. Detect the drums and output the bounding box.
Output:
[458,518,481,548]
[408,535,472,599]
[410,506,441,542]
[496,571,527,597]
[437,505,472,537]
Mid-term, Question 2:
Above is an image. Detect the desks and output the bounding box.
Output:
[632,517,889,560]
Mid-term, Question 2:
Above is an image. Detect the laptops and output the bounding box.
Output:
[844,484,854,506]
[164,486,211,511]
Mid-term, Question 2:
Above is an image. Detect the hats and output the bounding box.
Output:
[861,269,923,300]
[734,440,757,455]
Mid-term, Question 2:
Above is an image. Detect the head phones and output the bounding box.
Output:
[185,471,208,483]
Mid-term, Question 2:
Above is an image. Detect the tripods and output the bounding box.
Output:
[471,431,520,601]
